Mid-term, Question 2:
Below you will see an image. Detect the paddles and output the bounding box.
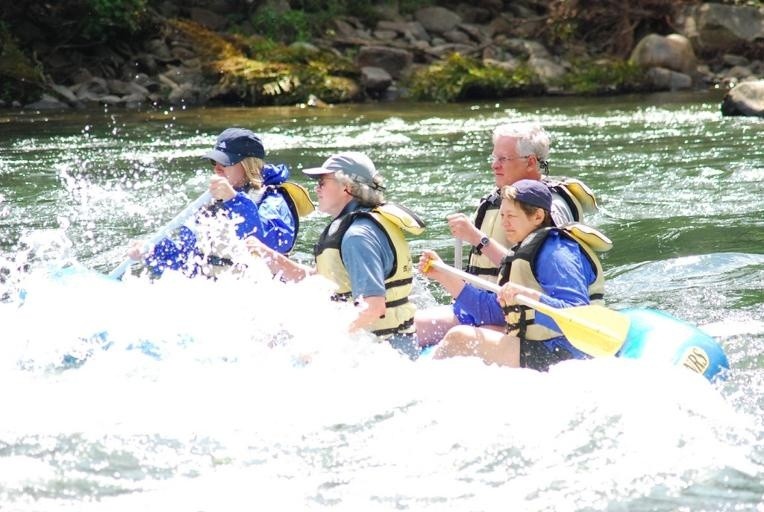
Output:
[420,255,631,357]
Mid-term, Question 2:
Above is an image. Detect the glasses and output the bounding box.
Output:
[319,176,335,186]
[489,154,527,163]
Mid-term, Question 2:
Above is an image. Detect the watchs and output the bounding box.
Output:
[476,236,490,251]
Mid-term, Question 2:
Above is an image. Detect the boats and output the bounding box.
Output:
[21,263,729,384]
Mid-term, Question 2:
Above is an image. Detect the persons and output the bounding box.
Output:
[239,149,426,360]
[429,179,603,373]
[126,127,319,287]
[414,119,597,348]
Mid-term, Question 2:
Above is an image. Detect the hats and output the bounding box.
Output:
[511,179,552,211]
[302,152,376,186]
[202,128,264,166]
